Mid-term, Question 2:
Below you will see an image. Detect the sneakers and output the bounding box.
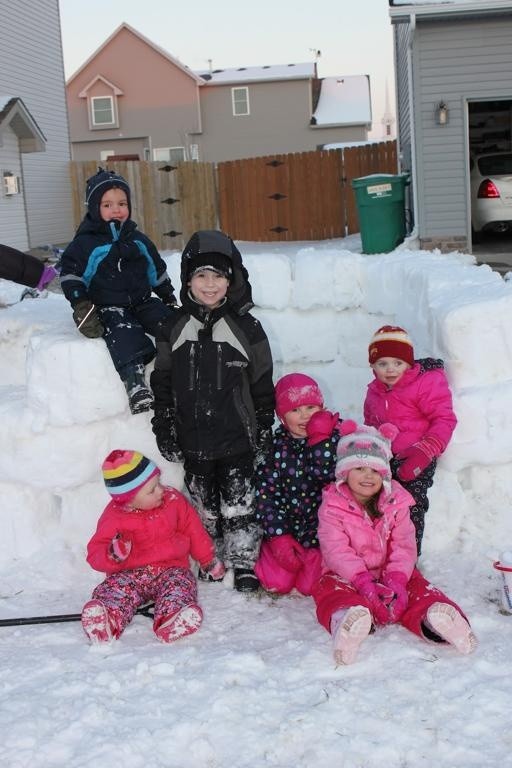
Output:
[81,599,117,642]
[427,602,480,656]
[155,604,203,644]
[332,605,371,665]
[234,568,259,592]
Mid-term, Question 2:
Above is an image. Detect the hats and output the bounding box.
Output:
[101,449,159,509]
[187,253,233,281]
[335,418,399,501]
[86,171,131,223]
[369,326,414,366]
[274,373,324,431]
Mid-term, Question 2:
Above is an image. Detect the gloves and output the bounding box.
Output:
[106,532,132,563]
[151,406,185,463]
[73,299,105,338]
[198,556,228,582]
[267,534,305,573]
[395,436,444,482]
[305,411,340,446]
[162,294,177,308]
[351,570,409,629]
[256,412,274,471]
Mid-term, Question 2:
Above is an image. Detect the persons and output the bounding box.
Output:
[249,372,355,595]
[311,420,480,666]
[59,166,177,415]
[80,448,225,644]
[360,325,458,560]
[149,230,276,593]
[0,242,64,297]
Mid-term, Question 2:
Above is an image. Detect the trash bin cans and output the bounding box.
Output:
[351,173,410,254]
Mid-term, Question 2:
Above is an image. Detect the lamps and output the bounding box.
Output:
[438,104,447,124]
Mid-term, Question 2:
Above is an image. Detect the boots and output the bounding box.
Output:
[124,363,155,415]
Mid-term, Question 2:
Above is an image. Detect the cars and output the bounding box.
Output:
[466,145,511,250]
